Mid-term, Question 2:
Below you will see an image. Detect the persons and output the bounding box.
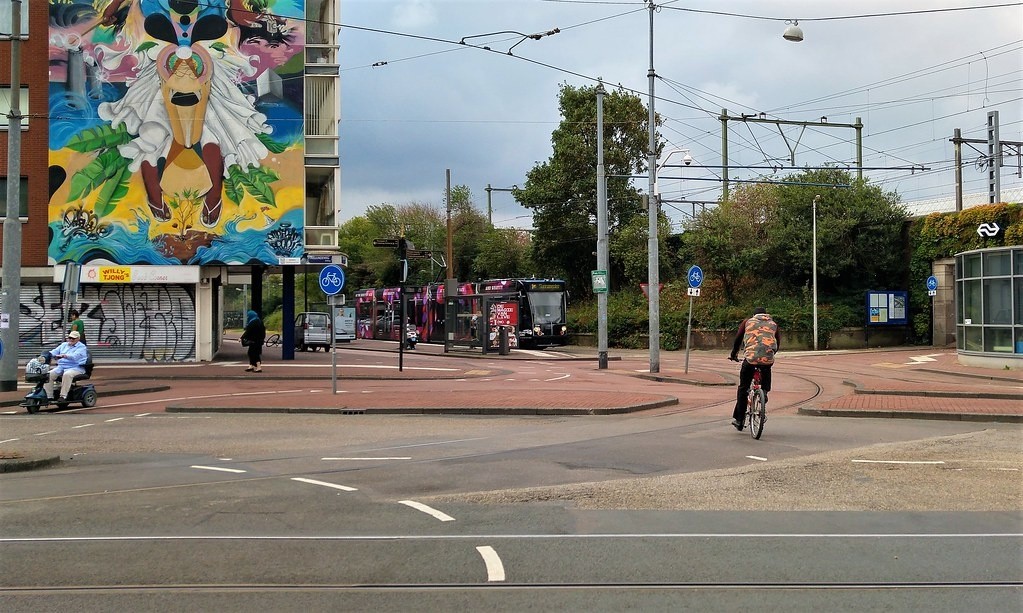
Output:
[69,309,85,335]
[730,308,781,431]
[238,310,266,372]
[44,331,88,403]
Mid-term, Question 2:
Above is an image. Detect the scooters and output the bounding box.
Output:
[406,323,419,350]
[18,349,99,413]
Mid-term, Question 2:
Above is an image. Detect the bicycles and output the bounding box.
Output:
[266,334,285,348]
[726,356,766,439]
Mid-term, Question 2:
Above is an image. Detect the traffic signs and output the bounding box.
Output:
[407,249,433,261]
[373,238,399,247]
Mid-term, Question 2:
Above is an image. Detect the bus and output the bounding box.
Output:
[351,277,571,350]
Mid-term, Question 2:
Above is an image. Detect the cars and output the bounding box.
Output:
[362,314,400,329]
[294,311,332,352]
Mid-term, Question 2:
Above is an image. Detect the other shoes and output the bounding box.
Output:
[47,398,53,401]
[245,368,254,372]
[254,370,262,373]
[763,416,768,423]
[732,420,745,431]
[58,397,65,401]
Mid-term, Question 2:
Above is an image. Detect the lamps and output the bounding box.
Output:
[783,19,803,42]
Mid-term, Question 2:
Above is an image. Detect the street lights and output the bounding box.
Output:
[812,194,822,353]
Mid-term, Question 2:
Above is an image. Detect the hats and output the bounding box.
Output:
[68,330,80,339]
